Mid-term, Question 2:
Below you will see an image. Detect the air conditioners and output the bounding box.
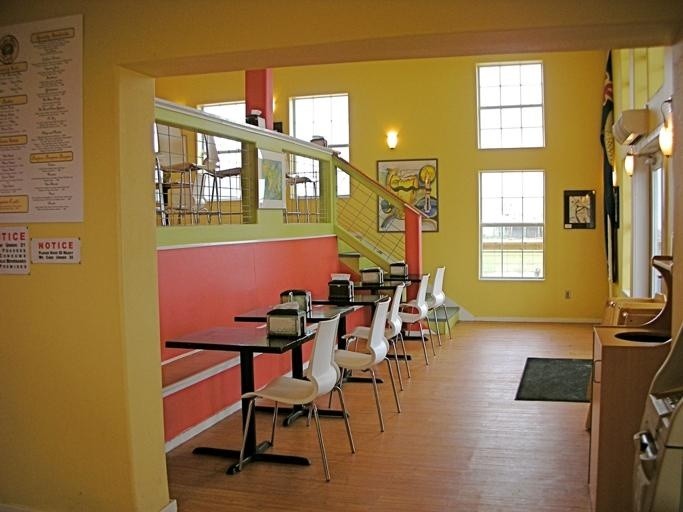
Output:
[609,108,659,146]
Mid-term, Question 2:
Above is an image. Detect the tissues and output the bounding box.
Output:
[359,266,384,284]
[389,260,408,277]
[266,302,307,338]
[280,289,312,312]
[328,273,355,300]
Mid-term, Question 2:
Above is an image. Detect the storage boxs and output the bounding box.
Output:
[235,304,364,426]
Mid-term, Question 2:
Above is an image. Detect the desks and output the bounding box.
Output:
[351,273,432,359]
[164,324,319,476]
[308,293,390,383]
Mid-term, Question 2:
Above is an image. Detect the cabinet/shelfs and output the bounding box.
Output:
[585,324,668,512]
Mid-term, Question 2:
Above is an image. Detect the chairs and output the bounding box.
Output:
[281,152,319,224]
[426,265,453,346]
[236,310,358,481]
[154,115,243,225]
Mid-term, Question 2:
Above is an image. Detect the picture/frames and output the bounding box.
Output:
[376,157,440,234]
[563,188,596,230]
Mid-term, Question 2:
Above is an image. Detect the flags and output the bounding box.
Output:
[600,49,618,283]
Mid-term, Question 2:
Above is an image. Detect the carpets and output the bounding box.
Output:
[513,353,593,403]
